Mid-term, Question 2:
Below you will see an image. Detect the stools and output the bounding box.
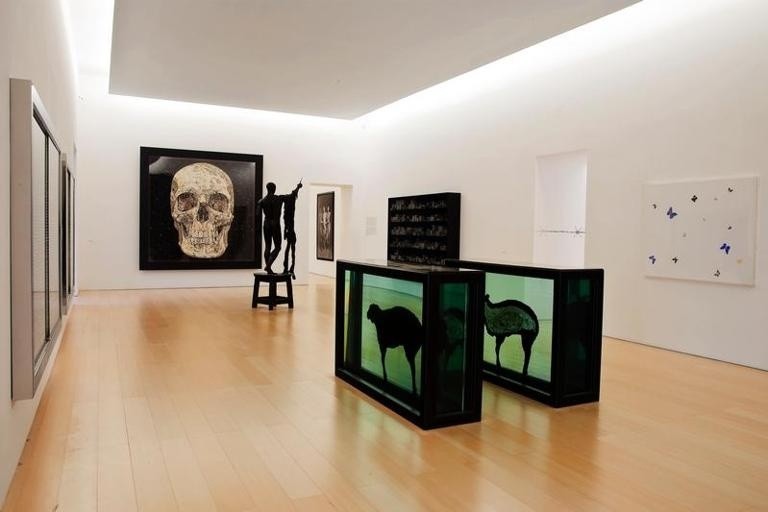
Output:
[250,272,294,310]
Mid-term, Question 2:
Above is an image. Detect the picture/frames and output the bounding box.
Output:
[316,190,335,260]
[138,145,263,271]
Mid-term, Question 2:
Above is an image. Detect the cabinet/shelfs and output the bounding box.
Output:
[384,192,463,265]
